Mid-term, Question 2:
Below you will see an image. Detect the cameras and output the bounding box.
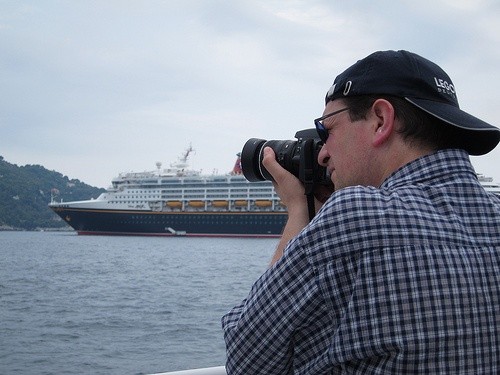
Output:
[241,127,335,204]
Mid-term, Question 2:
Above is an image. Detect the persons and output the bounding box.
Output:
[220,49,500,375]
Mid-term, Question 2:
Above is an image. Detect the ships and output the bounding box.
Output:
[47,147,500,239]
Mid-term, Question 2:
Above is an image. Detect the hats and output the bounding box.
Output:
[323,49,500,156]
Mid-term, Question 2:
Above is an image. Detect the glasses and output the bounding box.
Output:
[315,100,369,143]
